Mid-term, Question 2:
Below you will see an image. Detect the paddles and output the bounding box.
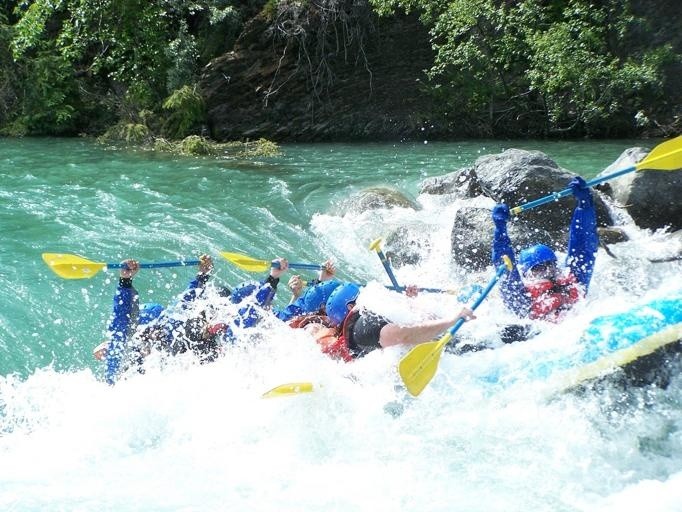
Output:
[41,254,206,279]
[510,136,681,216]
[220,252,329,271]
[399,255,513,397]
[261,382,322,399]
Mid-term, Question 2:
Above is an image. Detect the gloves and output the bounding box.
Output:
[568,176,591,200]
[491,203,511,223]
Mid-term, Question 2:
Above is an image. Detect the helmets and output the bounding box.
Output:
[304,279,360,327]
[518,244,559,273]
[231,280,260,304]
[138,302,163,324]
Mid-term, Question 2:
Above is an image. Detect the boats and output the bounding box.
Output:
[479,293,682,403]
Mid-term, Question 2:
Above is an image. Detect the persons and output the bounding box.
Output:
[491,178,600,329]
[106,253,336,386]
[285,278,477,364]
